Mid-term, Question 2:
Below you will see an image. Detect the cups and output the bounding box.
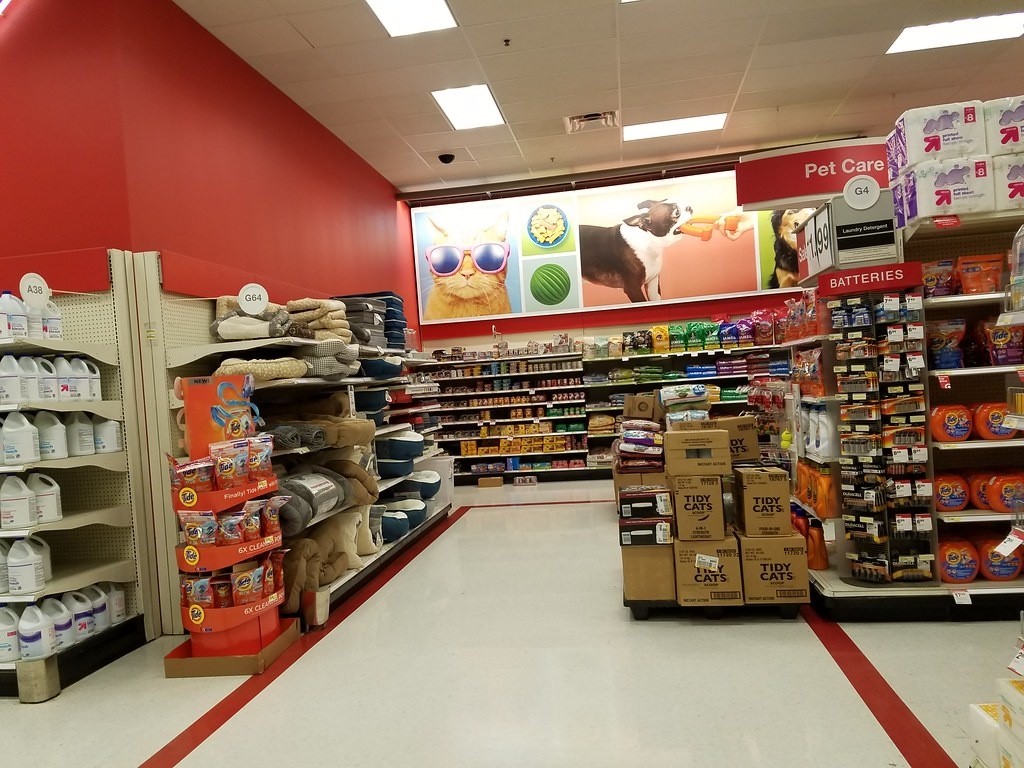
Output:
[443,434,455,440]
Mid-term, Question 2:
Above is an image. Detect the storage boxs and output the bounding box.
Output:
[619,518,674,545]
[618,486,675,518]
[641,472,670,487]
[733,467,793,537]
[672,476,725,541]
[673,419,719,431]
[614,472,641,508]
[735,530,811,603]
[652,389,667,429]
[478,476,503,487]
[674,525,744,607]
[623,397,654,419]
[622,544,677,601]
[714,414,761,461]
[664,428,733,477]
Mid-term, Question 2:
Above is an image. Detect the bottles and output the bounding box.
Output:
[791,503,828,570]
[0,352,101,404]
[1010,224,1024,312]
[801,403,838,459]
[0,410,122,465]
[0,291,62,343]
[797,457,839,519]
[0,469,63,529]
[0,582,126,663]
[0,535,52,594]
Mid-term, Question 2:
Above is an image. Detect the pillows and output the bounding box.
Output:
[325,460,380,504]
[359,453,381,482]
[317,446,368,466]
[277,537,319,614]
[345,504,387,556]
[333,511,364,569]
[319,551,350,583]
[304,517,341,591]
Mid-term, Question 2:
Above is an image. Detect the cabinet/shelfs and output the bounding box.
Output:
[0,208,1024,768]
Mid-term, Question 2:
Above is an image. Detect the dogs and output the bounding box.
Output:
[579,198,693,302]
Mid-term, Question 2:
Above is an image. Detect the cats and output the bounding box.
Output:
[421,213,512,320]
